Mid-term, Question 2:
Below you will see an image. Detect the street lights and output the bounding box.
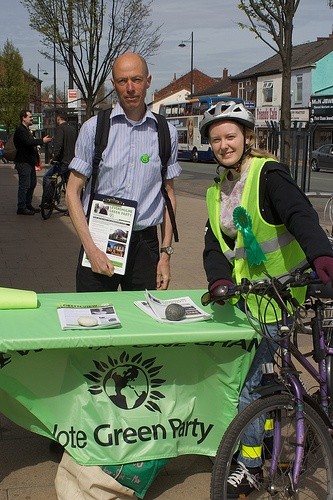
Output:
[178,40,194,97]
[38,70,48,110]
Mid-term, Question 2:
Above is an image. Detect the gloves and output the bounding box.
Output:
[210,279,234,305]
[313,256,333,288]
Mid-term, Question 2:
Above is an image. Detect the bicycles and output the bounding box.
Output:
[40,158,74,221]
[197,254,333,500]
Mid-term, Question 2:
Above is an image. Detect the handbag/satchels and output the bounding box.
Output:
[4,135,17,160]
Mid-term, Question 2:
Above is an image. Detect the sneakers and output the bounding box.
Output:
[227,461,263,498]
[233,436,273,459]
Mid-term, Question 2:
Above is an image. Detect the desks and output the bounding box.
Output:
[0,289,287,466]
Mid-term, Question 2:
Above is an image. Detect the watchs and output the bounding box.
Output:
[161,246,174,255]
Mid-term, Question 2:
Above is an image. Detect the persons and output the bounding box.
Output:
[0,139,7,164]
[13,110,52,215]
[65,52,183,292]
[38,111,78,208]
[198,101,333,500]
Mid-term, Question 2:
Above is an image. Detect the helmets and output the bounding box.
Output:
[199,100,255,139]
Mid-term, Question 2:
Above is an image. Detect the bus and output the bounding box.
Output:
[158,98,245,163]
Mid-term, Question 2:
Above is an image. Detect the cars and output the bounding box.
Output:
[310,145,333,172]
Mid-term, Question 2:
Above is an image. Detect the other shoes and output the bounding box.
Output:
[17,208,35,215]
[27,205,40,213]
[39,204,50,209]
